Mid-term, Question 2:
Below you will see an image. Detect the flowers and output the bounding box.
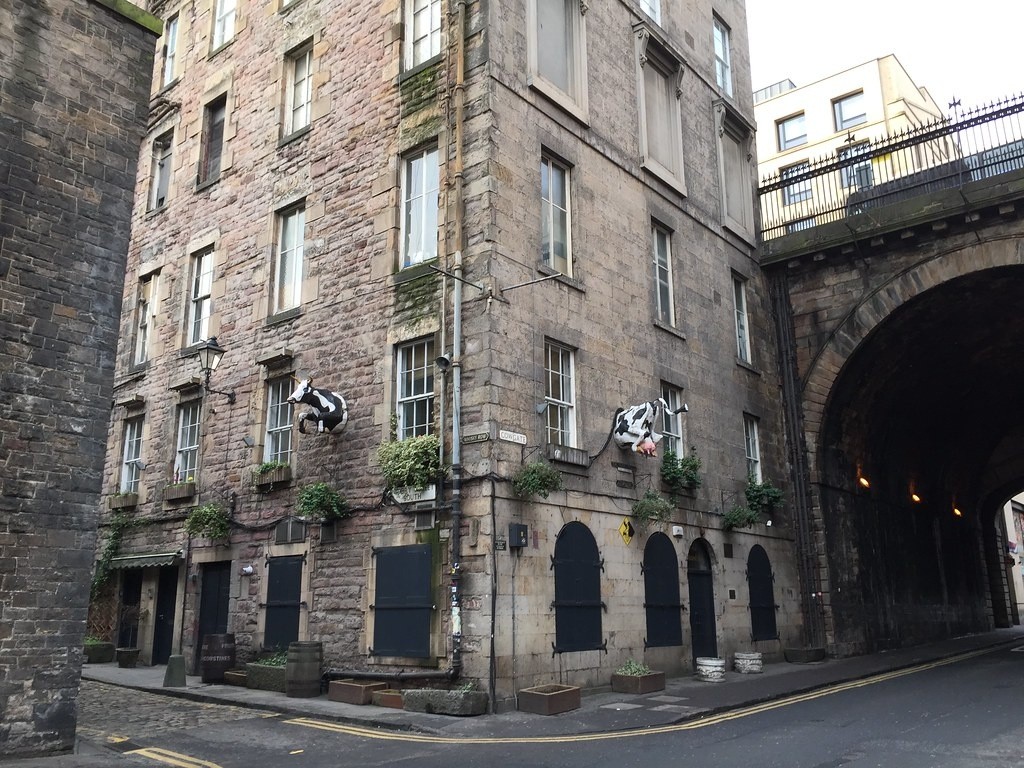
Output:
[164,478,196,487]
[507,456,564,508]
[632,489,681,533]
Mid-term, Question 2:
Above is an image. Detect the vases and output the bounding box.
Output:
[373,689,403,709]
[114,649,138,670]
[328,678,386,705]
[519,683,581,715]
[166,485,194,501]
[783,646,826,664]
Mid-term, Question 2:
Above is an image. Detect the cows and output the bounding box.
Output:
[612,397,688,457]
[287,375,343,435]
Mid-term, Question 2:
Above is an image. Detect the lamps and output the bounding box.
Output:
[196,335,236,405]
[858,477,872,497]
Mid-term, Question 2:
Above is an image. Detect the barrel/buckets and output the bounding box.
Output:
[200,633,236,683]
[285,641,321,696]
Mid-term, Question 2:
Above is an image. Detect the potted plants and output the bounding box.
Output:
[109,491,137,508]
[663,446,704,491]
[83,637,114,661]
[401,683,489,716]
[245,650,287,693]
[252,461,290,494]
[612,658,666,695]
